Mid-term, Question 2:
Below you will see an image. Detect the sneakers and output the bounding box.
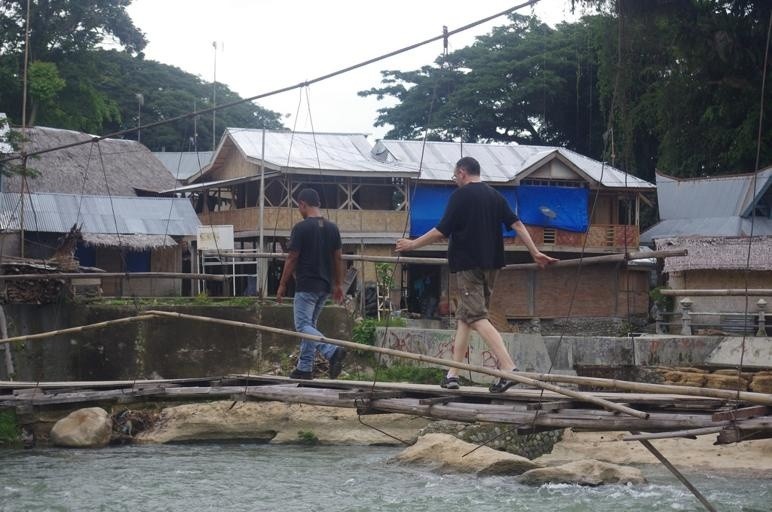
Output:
[488,368,523,393]
[288,367,313,379]
[440,374,460,389]
[328,347,348,379]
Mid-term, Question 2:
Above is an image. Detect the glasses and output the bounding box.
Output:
[451,168,465,181]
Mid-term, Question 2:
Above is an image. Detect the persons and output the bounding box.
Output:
[394,157,559,393]
[275,187,350,380]
